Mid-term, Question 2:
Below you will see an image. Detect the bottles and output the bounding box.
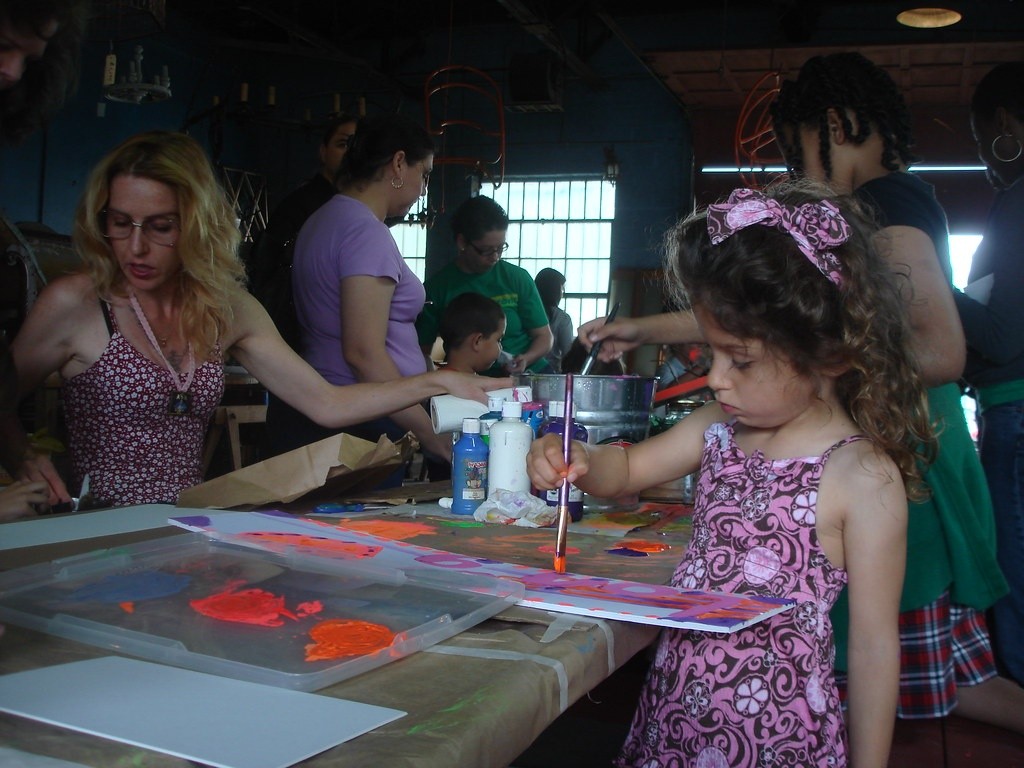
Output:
[479,399,503,444]
[452,418,489,515]
[538,401,589,522]
[489,402,531,495]
[663,400,704,430]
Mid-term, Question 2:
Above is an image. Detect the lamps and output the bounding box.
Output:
[896,0,964,28]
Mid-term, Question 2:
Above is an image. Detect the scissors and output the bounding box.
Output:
[314,502,392,514]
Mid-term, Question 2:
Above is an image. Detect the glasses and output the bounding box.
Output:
[461,231,508,256]
[97,210,181,247]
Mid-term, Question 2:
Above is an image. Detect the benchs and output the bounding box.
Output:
[889,714,1024,767]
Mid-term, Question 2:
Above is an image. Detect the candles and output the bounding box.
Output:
[334,92,340,113]
[358,96,366,116]
[267,85,275,107]
[303,108,310,120]
[240,82,249,102]
[212,96,220,108]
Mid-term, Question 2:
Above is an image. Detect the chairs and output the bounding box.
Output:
[0,229,83,437]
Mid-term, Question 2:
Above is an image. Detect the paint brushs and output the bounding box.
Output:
[554,373,576,573]
[579,303,619,376]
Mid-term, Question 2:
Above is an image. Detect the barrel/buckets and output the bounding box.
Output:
[517,374,656,513]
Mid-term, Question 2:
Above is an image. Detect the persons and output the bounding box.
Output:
[578,51,1023,735]
[268,112,457,494]
[0,0,78,527]
[523,178,939,768]
[415,195,553,377]
[528,265,572,373]
[0,112,514,511]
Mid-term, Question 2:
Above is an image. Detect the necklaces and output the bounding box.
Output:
[120,278,198,415]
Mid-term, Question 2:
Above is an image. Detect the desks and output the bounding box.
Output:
[0,480,695,768]
